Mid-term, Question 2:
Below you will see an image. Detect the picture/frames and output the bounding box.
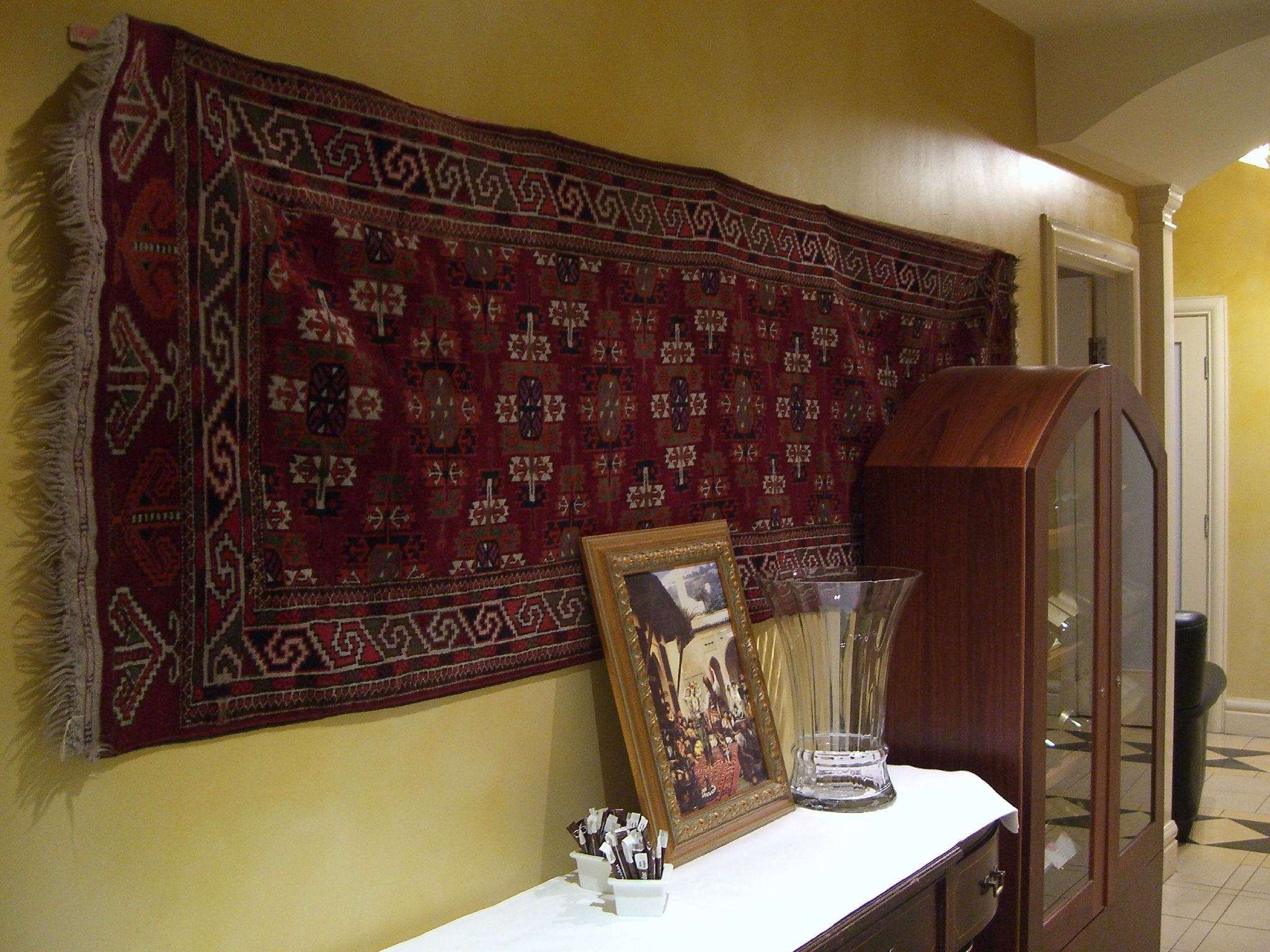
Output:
[579,520,795,868]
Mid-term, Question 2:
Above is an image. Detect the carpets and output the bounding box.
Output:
[33,9,1022,760]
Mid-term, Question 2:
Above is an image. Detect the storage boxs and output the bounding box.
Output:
[608,862,674,924]
[570,849,615,895]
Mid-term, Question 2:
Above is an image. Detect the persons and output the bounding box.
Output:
[655,666,765,806]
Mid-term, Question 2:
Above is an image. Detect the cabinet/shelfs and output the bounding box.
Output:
[378,764,1005,952]
[860,365,1167,952]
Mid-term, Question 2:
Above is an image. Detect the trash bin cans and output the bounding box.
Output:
[1174,611,1229,846]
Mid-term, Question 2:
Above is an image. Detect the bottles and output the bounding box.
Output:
[1047,564,1150,646]
[1046,475,1146,529]
[1046,652,1149,767]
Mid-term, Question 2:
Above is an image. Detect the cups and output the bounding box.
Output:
[756,566,925,813]
